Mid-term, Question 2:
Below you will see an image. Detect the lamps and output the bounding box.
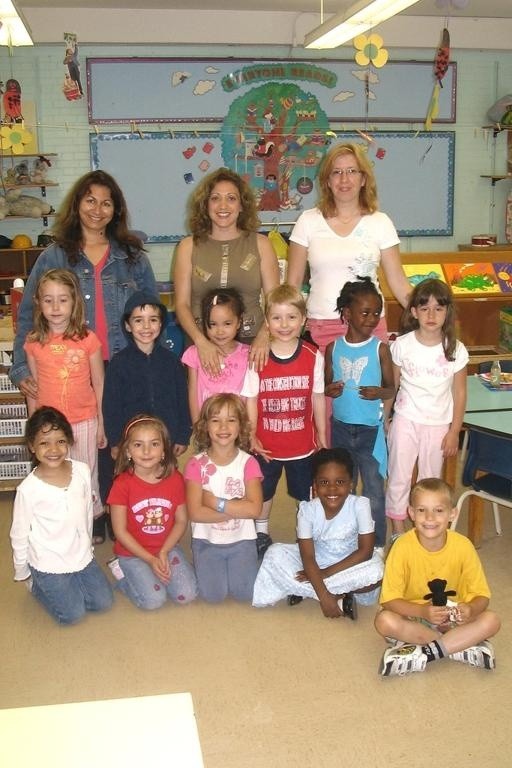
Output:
[0,0,35,50]
[298,0,420,50]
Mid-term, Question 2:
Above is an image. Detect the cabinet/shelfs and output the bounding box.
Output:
[0,153,61,221]
[1,247,49,314]
[377,249,511,376]
[1,340,30,493]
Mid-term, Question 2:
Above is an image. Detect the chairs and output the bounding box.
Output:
[448,426,512,538]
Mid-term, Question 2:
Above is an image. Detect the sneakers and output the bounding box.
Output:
[378,641,429,678]
[448,637,497,673]
[254,531,274,561]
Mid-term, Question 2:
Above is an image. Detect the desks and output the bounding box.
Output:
[441,373,512,552]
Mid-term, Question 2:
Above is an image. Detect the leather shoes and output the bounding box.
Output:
[340,594,357,621]
[286,594,303,607]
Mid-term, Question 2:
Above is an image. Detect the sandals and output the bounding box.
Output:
[91,512,117,546]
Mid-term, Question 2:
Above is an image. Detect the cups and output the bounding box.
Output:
[157,281,174,292]
[472,233,497,247]
[1,295,11,304]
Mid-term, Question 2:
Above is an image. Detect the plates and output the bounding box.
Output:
[479,372,511,385]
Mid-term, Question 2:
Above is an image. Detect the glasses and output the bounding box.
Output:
[330,168,359,177]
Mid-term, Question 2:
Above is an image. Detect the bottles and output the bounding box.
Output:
[490,360,501,388]
